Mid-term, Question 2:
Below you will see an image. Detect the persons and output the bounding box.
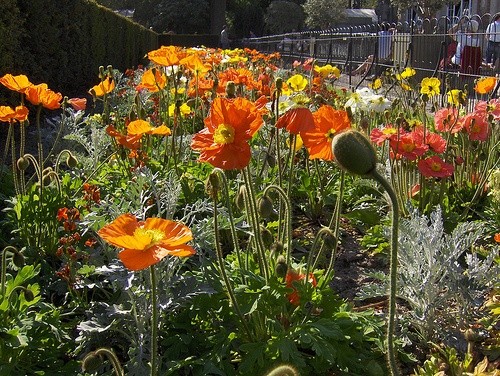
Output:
[220,24,232,49]
[376,22,394,61]
[485,14,500,67]
[448,17,483,72]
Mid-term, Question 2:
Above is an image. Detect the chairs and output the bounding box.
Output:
[460,45,483,80]
